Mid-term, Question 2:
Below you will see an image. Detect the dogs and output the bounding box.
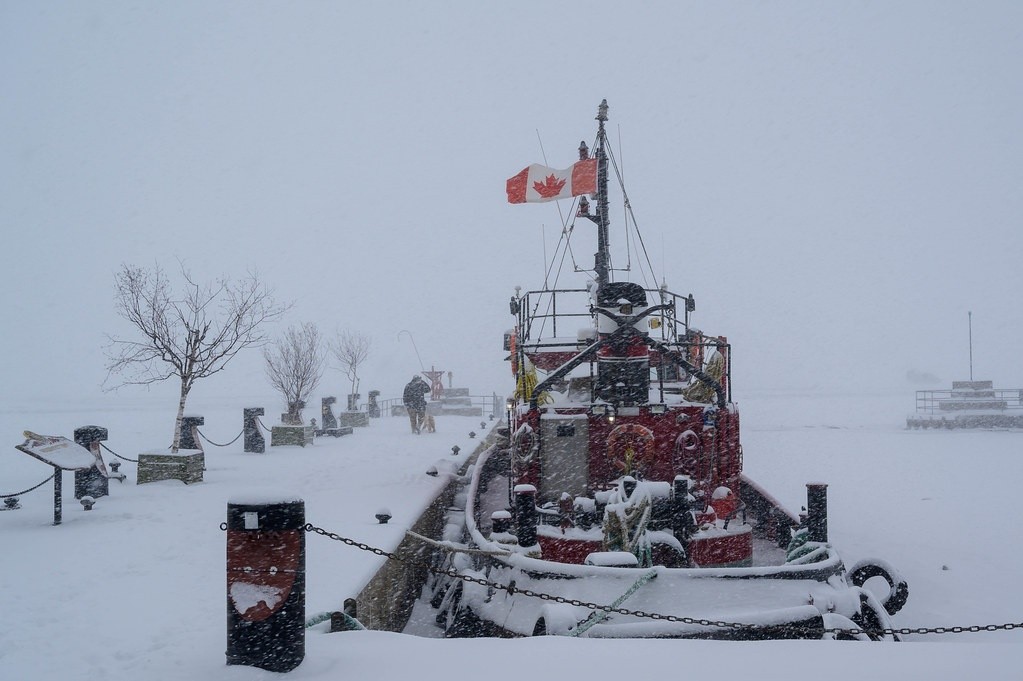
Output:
[420,416,436,433]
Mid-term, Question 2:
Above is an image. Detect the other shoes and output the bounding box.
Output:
[416,427,421,435]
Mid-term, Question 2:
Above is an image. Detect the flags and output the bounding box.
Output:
[505,157,597,204]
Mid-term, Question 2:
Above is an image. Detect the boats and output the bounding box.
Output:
[445,99,900,640]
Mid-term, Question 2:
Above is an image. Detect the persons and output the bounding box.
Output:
[402,375,432,434]
